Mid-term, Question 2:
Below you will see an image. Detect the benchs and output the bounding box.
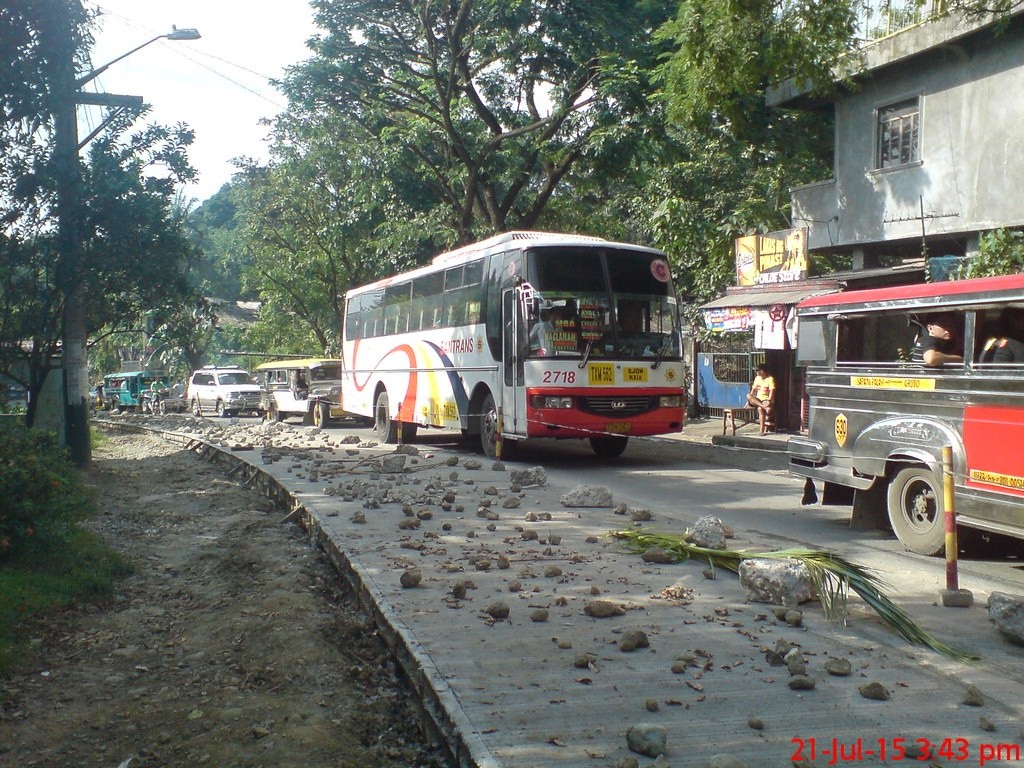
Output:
[723,407,779,435]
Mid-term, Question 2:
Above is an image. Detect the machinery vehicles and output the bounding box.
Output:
[141,387,187,415]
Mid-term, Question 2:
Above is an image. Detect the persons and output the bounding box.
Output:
[521,306,564,356]
[296,371,308,399]
[978,306,1024,369]
[151,377,165,415]
[912,311,963,366]
[747,364,777,436]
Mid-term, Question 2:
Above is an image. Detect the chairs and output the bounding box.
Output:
[978,337,1001,370]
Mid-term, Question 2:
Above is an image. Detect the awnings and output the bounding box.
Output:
[698,289,839,309]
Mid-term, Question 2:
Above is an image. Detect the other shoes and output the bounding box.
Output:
[759,432,765,436]
[765,407,773,416]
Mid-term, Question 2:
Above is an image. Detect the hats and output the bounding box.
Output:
[751,363,768,372]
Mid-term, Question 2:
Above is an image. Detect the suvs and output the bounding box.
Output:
[187,365,267,418]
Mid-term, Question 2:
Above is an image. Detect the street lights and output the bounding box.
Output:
[54,28,202,467]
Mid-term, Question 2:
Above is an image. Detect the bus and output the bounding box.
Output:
[255,357,374,428]
[340,231,688,462]
[103,370,171,412]
[785,272,1024,557]
